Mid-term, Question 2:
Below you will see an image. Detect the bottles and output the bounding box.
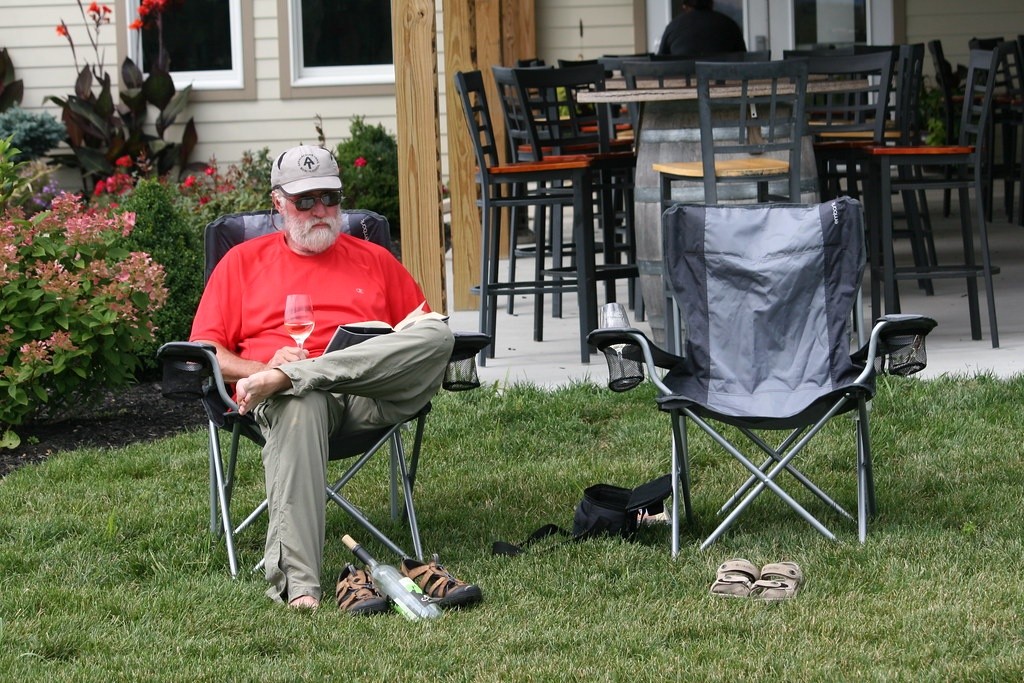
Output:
[341,533,445,625]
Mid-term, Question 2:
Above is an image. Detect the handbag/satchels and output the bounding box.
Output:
[492,473,673,558]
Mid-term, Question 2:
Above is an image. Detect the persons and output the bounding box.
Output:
[187,145,455,608]
[658,0,748,57]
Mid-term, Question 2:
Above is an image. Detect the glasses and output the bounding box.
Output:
[274,190,342,211]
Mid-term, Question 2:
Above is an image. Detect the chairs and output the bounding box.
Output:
[455,36,1024,367]
[156,210,490,582]
[588,198,939,562]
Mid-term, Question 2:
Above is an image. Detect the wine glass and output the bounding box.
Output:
[285,293,315,350]
[597,303,639,388]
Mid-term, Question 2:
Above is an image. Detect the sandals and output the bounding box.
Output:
[401,553,482,611]
[335,564,388,615]
[710,558,760,598]
[750,562,803,605]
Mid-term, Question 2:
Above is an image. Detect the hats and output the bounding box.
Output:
[271,146,342,196]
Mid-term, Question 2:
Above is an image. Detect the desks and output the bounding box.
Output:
[578,77,869,322]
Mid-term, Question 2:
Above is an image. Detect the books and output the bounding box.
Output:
[323,299,450,356]
